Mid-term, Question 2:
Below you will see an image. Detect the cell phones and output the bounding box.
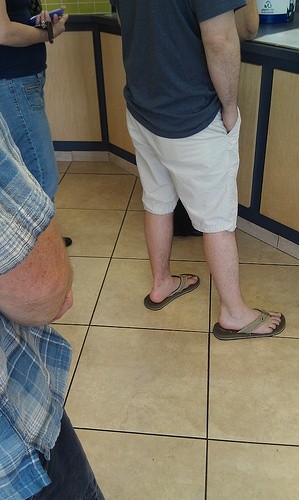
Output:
[30,8,63,27]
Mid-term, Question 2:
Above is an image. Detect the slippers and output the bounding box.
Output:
[144,274,200,311]
[213,307,285,341]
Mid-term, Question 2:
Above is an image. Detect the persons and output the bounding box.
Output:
[0,0,72,248]
[0,117,106,500]
[116,0,288,340]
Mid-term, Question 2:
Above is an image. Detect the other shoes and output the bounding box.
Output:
[174,229,202,237]
[62,238,72,247]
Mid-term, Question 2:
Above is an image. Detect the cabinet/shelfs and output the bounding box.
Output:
[44,16,299,260]
